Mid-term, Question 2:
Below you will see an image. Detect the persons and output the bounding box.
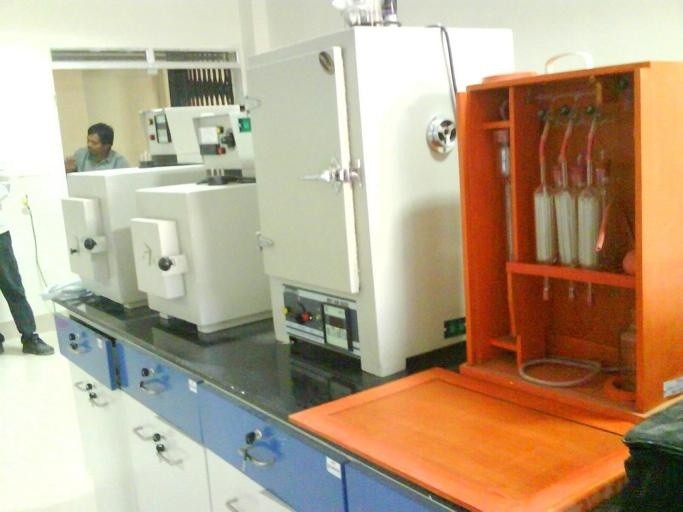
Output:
[63,121,131,176]
[0,213,55,356]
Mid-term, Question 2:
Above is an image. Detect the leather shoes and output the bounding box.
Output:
[0,342,2,353]
[22,334,53,356]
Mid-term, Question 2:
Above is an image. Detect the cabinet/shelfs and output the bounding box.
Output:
[46,289,679,510]
[456,61,683,445]
